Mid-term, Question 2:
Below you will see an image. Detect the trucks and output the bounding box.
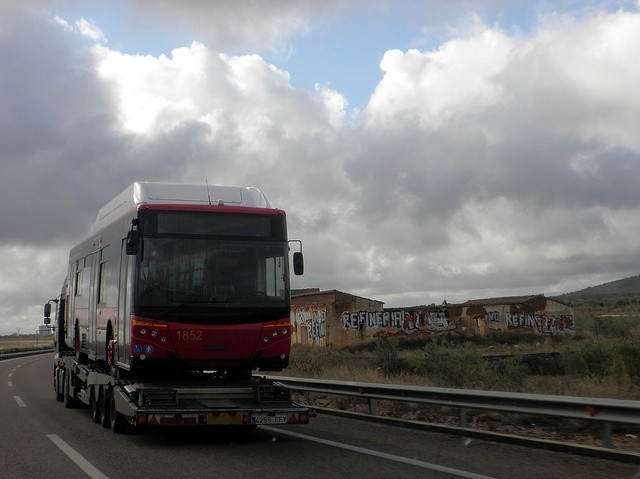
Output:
[44,276,316,433]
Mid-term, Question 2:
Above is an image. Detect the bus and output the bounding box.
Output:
[65,182,303,382]
